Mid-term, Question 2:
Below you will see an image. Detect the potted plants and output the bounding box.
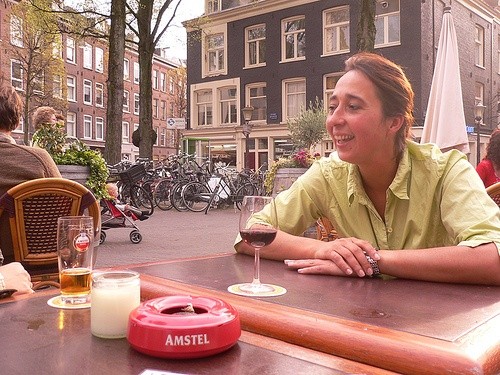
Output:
[29,119,118,228]
[262,94,332,233]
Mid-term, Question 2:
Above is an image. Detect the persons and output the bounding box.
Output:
[106,183,150,221]
[31,106,66,151]
[234,52,500,285]
[475,129,500,208]
[0,249,36,298]
[0,84,62,269]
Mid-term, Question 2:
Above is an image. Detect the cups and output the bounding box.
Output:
[57,216,94,305]
[91,271,140,340]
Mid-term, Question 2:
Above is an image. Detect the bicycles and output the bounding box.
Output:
[111,150,268,216]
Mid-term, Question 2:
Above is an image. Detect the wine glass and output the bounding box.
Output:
[238,196,279,294]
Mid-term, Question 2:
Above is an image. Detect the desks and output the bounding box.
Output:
[0,284,403,375]
[91,250,500,375]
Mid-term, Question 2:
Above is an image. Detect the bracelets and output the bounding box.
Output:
[0,274,6,290]
[362,249,381,275]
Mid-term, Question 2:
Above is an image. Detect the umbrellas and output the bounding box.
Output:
[420,6,471,156]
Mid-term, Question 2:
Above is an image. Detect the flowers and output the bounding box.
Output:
[289,150,322,168]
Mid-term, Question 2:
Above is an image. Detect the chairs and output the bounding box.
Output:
[0,178,100,292]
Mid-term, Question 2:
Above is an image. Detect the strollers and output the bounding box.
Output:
[99,182,142,245]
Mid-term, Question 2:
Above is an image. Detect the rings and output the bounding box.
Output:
[30,283,32,288]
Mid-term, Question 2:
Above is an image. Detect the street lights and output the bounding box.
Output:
[471,100,487,165]
[241,104,254,173]
